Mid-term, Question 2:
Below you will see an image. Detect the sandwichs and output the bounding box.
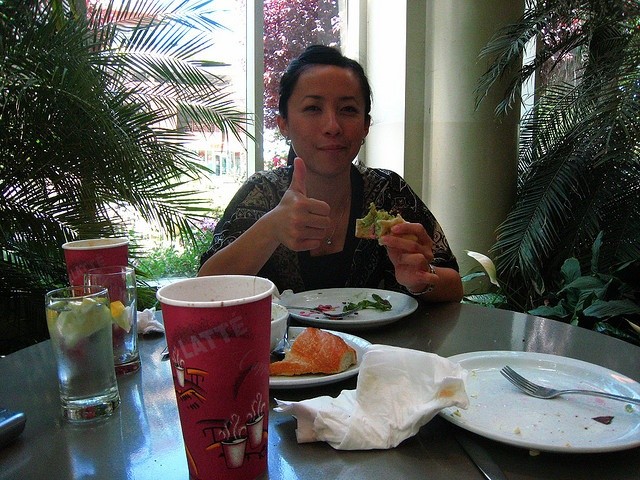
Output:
[356,202,419,247]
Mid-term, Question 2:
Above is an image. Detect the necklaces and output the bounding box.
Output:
[318,177,352,246]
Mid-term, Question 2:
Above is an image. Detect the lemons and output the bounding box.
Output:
[109,300,130,332]
[56,297,105,345]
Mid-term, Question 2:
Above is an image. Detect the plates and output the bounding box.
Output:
[282,287,419,327]
[268,326,374,389]
[435,351,640,453]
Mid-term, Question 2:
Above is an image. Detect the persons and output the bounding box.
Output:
[199,45,463,302]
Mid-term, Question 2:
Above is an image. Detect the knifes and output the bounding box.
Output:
[449,422,508,480]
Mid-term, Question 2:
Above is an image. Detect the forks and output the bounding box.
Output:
[499,365,640,408]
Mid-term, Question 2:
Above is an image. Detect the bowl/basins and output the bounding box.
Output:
[271,303,288,351]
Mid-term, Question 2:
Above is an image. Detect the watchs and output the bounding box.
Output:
[405,263,437,296]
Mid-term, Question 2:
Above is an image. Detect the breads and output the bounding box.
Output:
[270,327,357,375]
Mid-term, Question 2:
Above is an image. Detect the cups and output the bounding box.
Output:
[175,365,185,387]
[155,275,275,480]
[84,265,141,379]
[61,238,140,373]
[45,286,122,423]
[245,412,265,450]
[219,434,246,469]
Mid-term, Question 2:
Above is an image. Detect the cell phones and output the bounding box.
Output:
[0,405,27,449]
